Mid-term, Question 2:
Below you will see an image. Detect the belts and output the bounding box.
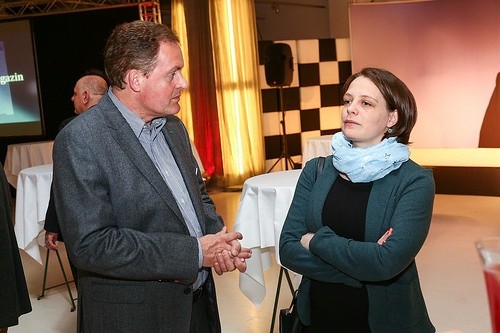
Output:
[192,278,207,303]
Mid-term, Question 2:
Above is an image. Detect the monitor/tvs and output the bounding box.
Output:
[0,16,48,141]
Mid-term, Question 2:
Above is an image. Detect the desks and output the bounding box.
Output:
[301,135,333,168]
[4,141,55,190]
[234,168,303,304]
[14,164,78,312]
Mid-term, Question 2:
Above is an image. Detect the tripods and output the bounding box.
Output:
[266,86,295,173]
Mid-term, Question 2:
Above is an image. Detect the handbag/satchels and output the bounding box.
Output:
[279,308,295,333]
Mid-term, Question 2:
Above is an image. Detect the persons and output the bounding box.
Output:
[44,75,106,293]
[52,20,254,333]
[279,68,437,333]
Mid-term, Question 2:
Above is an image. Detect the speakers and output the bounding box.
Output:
[263,43,293,86]
